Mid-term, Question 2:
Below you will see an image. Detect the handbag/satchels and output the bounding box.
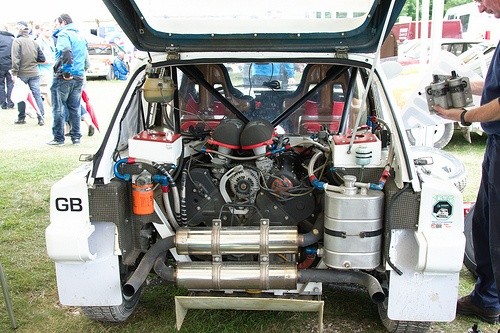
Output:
[10,77,30,103]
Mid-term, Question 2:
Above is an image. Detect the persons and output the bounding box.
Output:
[252,60,295,91]
[113,51,127,80]
[46,14,95,147]
[0,25,15,110]
[11,21,46,126]
[431,0,500,324]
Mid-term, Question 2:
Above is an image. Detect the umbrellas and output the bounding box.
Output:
[8,70,44,120]
[82,91,101,134]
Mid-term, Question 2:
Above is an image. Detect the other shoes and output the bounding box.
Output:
[65,133,71,136]
[72,139,80,144]
[14,119,26,124]
[457,296,500,324]
[46,140,65,145]
[38,120,44,126]
[2,106,7,109]
[88,125,95,136]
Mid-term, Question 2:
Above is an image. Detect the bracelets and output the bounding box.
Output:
[460,110,472,126]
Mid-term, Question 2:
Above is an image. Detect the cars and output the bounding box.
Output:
[45,0,500,333]
